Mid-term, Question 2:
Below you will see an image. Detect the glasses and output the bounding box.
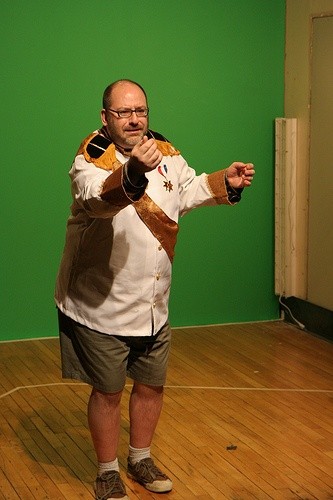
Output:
[106,106,149,118]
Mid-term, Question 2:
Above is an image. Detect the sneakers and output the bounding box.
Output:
[95,454,173,499]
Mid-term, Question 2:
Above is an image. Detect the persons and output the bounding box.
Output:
[53,77,256,500]
